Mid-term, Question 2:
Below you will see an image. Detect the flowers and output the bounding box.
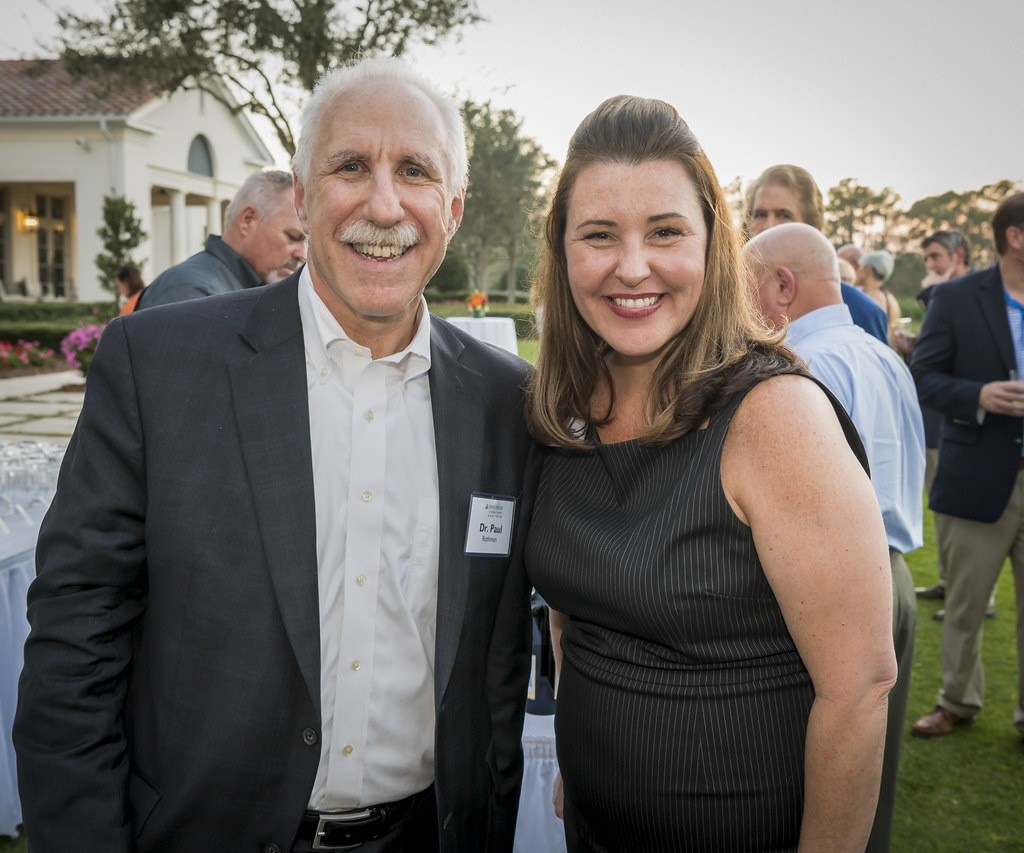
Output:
[466,290,489,318]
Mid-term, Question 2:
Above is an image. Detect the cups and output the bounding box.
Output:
[893,317,912,348]
[1009,370,1024,404]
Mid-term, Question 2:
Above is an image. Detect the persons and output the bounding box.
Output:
[743,166,1024,852]
[138,171,302,311]
[529,94,893,852]
[11,54,539,853]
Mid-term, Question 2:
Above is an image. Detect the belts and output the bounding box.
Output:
[296,804,410,850]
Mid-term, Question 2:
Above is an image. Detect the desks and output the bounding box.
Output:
[446,316,518,360]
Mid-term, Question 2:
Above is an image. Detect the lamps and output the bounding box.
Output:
[21,210,39,232]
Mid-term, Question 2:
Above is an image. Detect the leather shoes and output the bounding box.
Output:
[911,705,976,737]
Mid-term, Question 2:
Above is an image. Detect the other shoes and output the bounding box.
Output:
[914,584,944,599]
[932,606,995,621]
